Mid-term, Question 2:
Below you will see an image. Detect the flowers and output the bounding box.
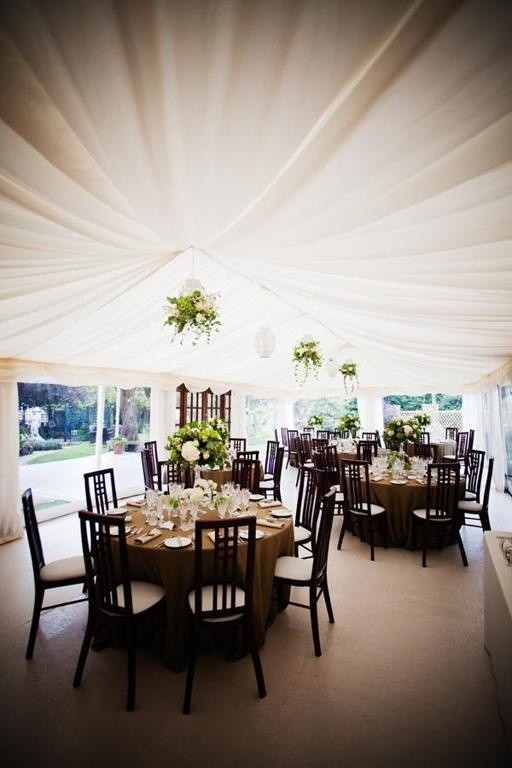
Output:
[165,422,228,473]
[163,280,224,350]
[308,413,434,444]
[292,335,324,386]
[339,362,362,396]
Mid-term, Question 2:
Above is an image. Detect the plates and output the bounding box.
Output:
[163,535,191,549]
[107,506,127,514]
[250,492,265,500]
[108,524,130,535]
[238,528,264,540]
[271,507,292,516]
[390,480,407,485]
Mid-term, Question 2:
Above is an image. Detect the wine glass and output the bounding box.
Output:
[142,481,250,530]
